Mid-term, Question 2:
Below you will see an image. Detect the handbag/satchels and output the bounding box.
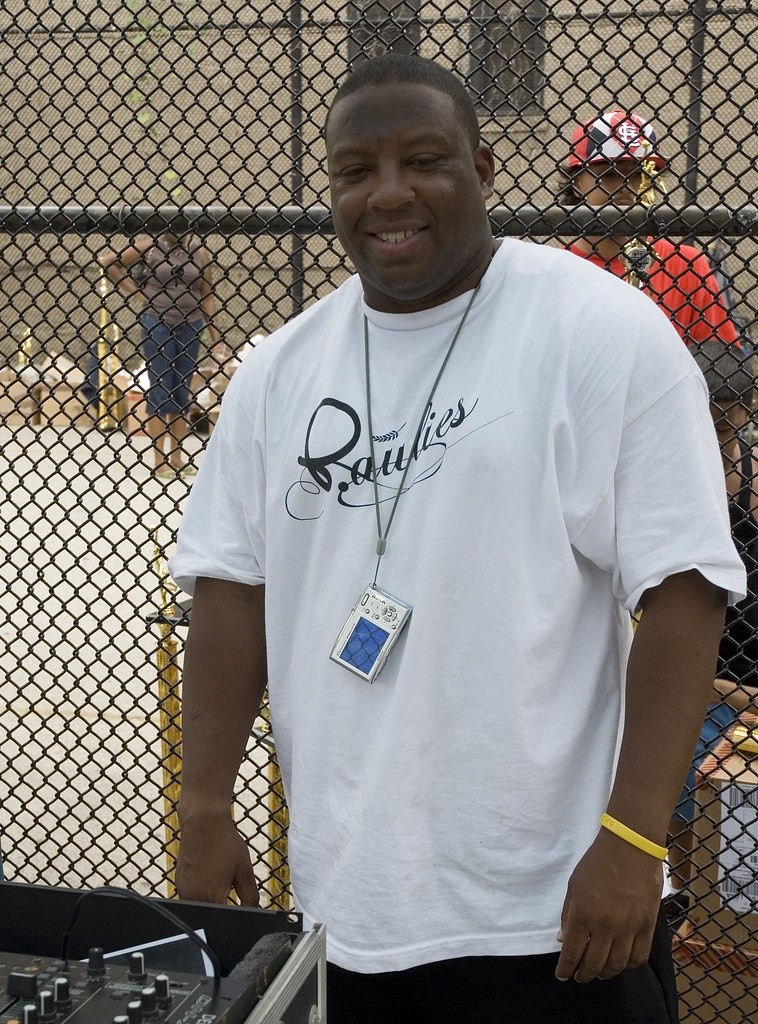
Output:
[132,237,155,286]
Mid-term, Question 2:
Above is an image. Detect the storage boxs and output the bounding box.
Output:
[673,710,758,1024]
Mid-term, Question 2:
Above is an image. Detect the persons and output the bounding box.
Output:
[551,115,758,895]
[173,51,749,1024]
[102,190,229,481]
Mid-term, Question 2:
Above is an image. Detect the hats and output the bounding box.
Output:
[569,109,673,173]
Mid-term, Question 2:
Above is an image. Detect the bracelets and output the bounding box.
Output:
[597,810,669,861]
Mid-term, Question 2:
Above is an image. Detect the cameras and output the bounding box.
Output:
[329,583,412,684]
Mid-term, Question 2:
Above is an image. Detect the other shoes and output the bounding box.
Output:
[661,894,690,919]
[182,464,197,476]
[157,465,186,480]
